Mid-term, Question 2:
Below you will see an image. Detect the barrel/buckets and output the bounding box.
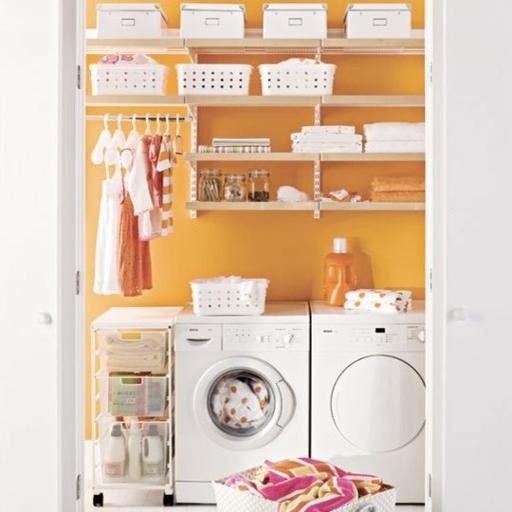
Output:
[101,423,128,480]
[141,424,164,475]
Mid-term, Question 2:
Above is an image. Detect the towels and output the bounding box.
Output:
[362,119,426,155]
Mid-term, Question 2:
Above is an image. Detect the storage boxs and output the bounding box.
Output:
[342,1,412,39]
[261,2,329,39]
[95,369,170,418]
[98,418,169,485]
[179,3,249,39]
[99,330,169,375]
[94,2,169,39]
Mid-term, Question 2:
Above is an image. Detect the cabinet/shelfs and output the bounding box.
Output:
[89,305,185,505]
[86,35,428,211]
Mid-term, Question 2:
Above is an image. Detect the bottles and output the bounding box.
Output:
[102,416,165,479]
[198,166,272,203]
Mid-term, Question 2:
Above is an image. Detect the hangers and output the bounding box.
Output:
[103,112,181,136]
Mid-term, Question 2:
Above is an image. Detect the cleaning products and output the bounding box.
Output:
[321,236,358,306]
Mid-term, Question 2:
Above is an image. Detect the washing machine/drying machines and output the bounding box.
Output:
[310,298,426,505]
[172,303,310,505]
